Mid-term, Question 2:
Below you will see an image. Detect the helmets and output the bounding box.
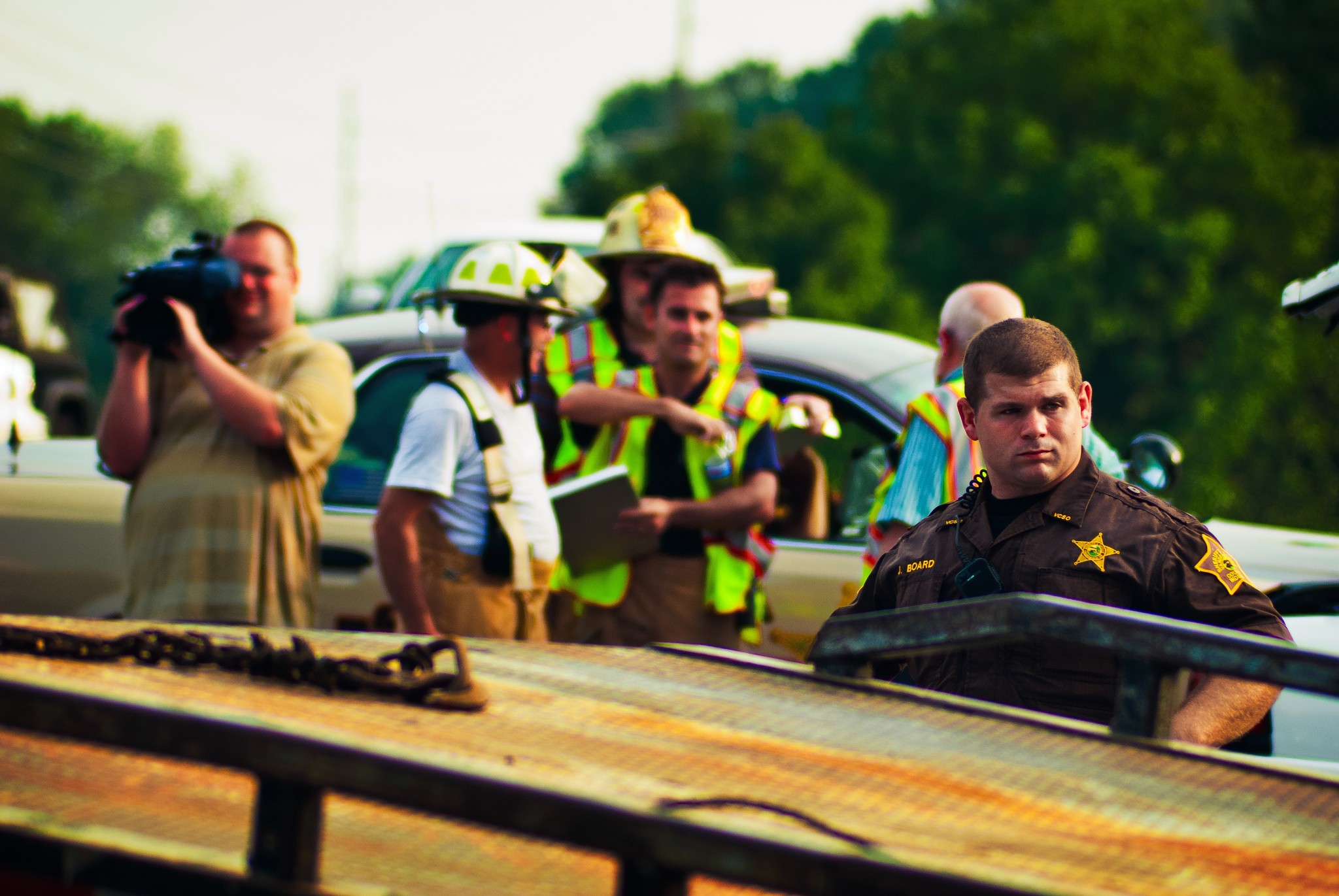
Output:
[411,239,577,316]
[584,184,728,267]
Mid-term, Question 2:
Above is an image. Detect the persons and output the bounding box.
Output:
[859,281,1025,594]
[94,219,354,630]
[528,184,832,652]
[371,240,579,642]
[850,317,1297,747]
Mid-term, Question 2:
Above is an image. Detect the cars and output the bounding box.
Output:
[3,307,1185,662]
[379,216,748,312]
